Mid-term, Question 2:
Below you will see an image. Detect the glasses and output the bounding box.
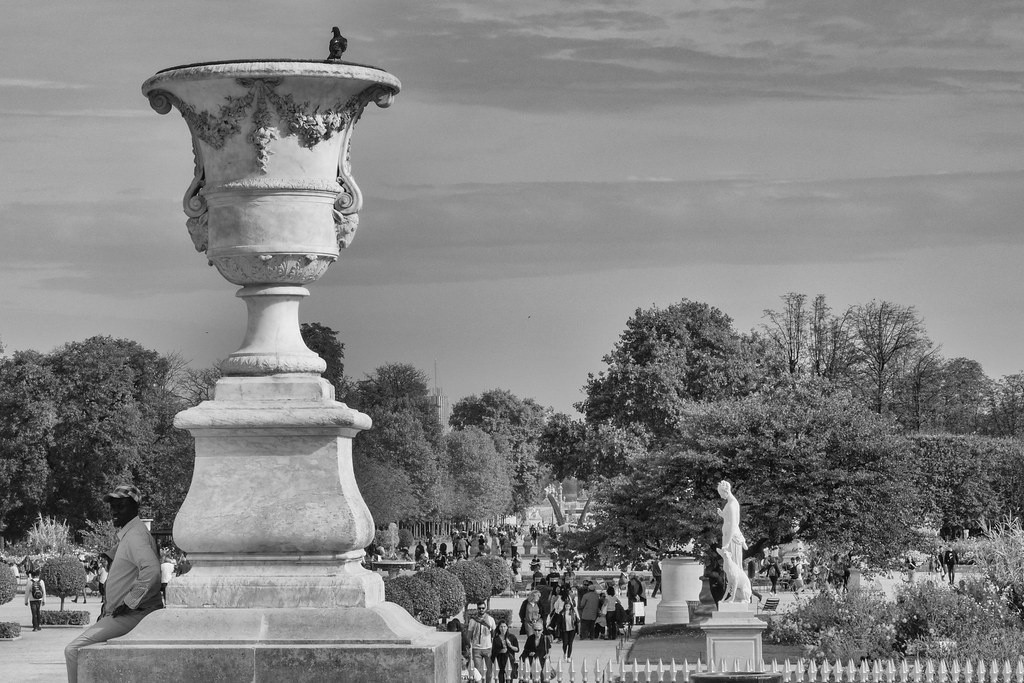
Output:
[478,608,485,610]
[534,629,542,632]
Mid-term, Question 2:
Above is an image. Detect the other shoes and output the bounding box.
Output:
[563,653,567,661]
[567,657,571,663]
[83,601,86,603]
[71,599,77,603]
[33,627,41,631]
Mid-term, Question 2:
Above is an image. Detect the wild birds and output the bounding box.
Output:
[324,25,348,61]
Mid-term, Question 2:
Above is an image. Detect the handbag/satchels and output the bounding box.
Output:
[633,598,645,617]
[601,603,608,617]
[513,662,519,680]
[550,667,556,679]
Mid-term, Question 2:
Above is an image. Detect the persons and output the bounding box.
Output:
[364,522,959,683]
[6,545,177,632]
[717,480,748,571]
[64,484,163,683]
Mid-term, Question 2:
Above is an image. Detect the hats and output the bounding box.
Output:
[102,484,143,506]
[587,585,596,591]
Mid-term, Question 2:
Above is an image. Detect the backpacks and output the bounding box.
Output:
[30,578,43,599]
